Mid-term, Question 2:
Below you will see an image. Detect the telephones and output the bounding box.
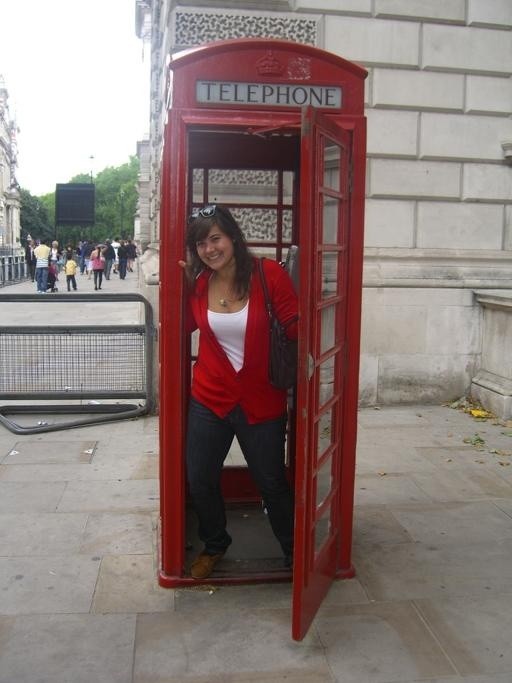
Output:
[279,262,286,267]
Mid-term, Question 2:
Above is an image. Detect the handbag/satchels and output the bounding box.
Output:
[258,256,299,392]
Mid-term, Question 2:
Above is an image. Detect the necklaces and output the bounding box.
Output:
[213,273,229,307]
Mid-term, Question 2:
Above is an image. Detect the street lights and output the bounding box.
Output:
[120,190,126,239]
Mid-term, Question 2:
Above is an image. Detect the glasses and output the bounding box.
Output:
[186,205,221,225]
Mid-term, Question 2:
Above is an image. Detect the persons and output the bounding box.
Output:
[177,204,300,580]
[25,237,137,293]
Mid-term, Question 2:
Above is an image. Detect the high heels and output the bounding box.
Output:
[190,548,225,579]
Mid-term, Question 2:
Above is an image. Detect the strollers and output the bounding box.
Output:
[45,266,59,293]
